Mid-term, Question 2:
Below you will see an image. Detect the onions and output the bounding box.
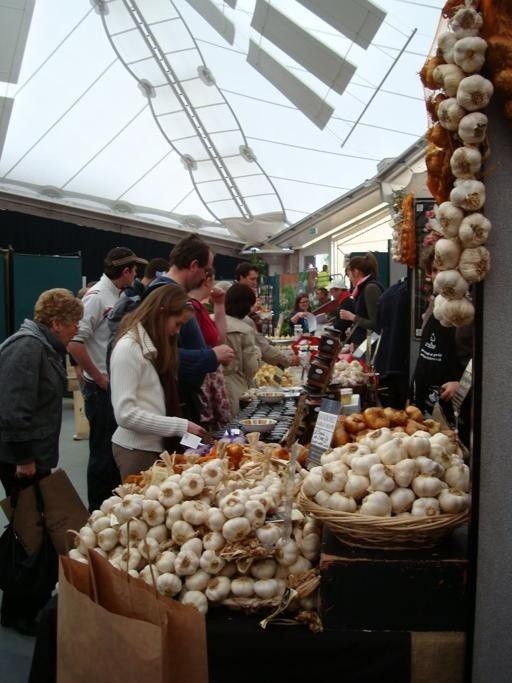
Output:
[488,37,512,121]
[333,405,456,446]
[420,57,450,204]
[400,212,415,264]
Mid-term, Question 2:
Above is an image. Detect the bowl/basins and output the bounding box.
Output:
[255,392,285,404]
[239,419,277,433]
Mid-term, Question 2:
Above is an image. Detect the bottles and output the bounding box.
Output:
[255,319,304,340]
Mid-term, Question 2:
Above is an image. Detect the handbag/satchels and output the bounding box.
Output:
[0,468,91,558]
[55,521,211,683]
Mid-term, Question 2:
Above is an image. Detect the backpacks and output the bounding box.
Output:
[0,528,59,596]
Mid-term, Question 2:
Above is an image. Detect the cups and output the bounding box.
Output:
[341,388,353,404]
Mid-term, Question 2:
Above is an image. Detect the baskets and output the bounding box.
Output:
[301,443,472,550]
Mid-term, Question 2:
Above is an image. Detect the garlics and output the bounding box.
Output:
[68,427,471,615]
[432,8,495,330]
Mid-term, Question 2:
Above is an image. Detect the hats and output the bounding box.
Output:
[103,246,149,267]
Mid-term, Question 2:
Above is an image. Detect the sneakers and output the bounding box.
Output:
[1,613,44,640]
